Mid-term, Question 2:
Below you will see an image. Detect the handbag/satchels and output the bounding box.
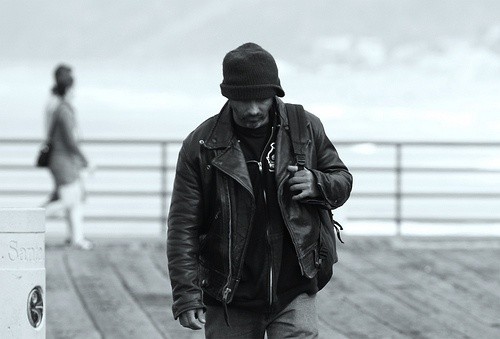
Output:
[285,102,337,291]
[38,144,52,166]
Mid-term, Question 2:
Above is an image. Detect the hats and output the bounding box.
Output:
[220,42,284,97]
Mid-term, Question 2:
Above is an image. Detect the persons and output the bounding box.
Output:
[165,42,353,339]
[36,64,93,251]
